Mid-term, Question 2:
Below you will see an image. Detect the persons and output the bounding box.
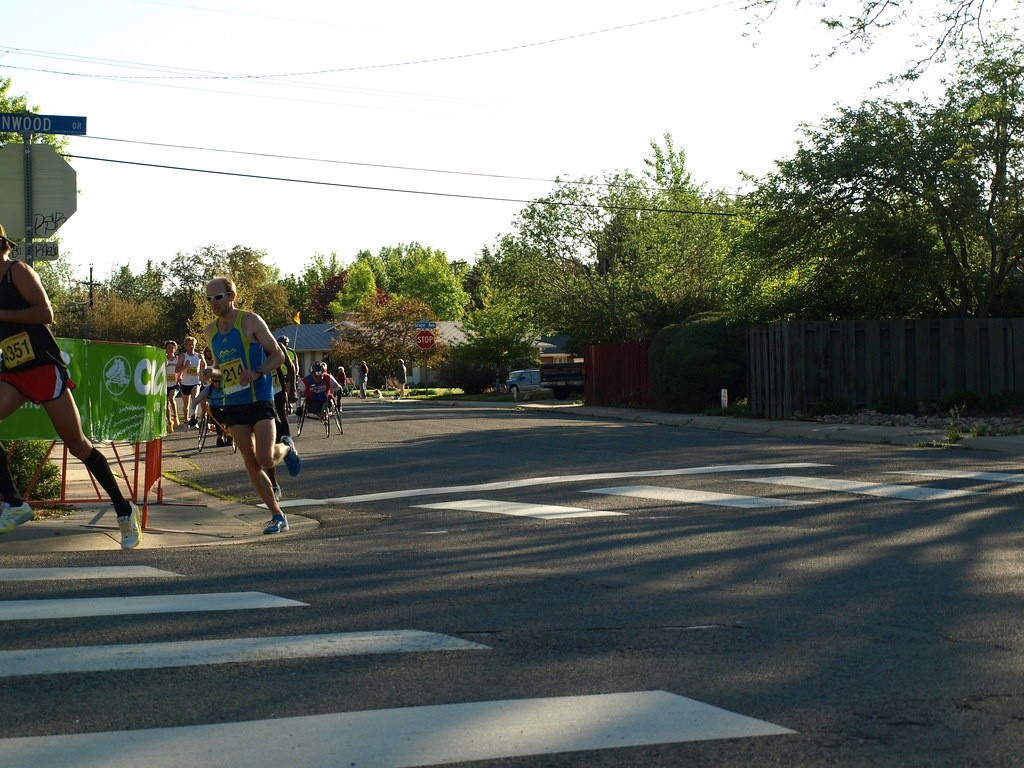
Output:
[358,361,369,398]
[0,224,142,551]
[397,359,407,399]
[198,277,302,535]
[164,336,346,501]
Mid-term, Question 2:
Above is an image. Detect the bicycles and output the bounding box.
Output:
[198,405,237,453]
[296,398,344,437]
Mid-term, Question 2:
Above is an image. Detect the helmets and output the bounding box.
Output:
[277,335,290,344]
[211,369,221,380]
[309,361,327,377]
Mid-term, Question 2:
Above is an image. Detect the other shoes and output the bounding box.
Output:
[168,422,174,433]
[216,436,225,447]
[173,416,179,426]
[224,435,233,446]
[274,484,282,502]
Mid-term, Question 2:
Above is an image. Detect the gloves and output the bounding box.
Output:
[295,407,303,419]
[188,414,198,427]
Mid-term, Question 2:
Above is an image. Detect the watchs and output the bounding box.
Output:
[255,367,263,376]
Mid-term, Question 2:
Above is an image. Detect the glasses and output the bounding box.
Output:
[206,291,232,303]
[214,379,221,383]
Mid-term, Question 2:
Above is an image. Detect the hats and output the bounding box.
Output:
[0,224,18,247]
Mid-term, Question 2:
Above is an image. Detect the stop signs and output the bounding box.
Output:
[415,330,434,350]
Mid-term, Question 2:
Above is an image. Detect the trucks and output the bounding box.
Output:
[540,363,584,399]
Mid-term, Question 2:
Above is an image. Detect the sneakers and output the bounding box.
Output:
[280,435,301,477]
[0,501,34,535]
[117,501,142,550]
[262,513,289,534]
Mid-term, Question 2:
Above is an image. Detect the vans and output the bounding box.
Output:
[505,370,542,393]
